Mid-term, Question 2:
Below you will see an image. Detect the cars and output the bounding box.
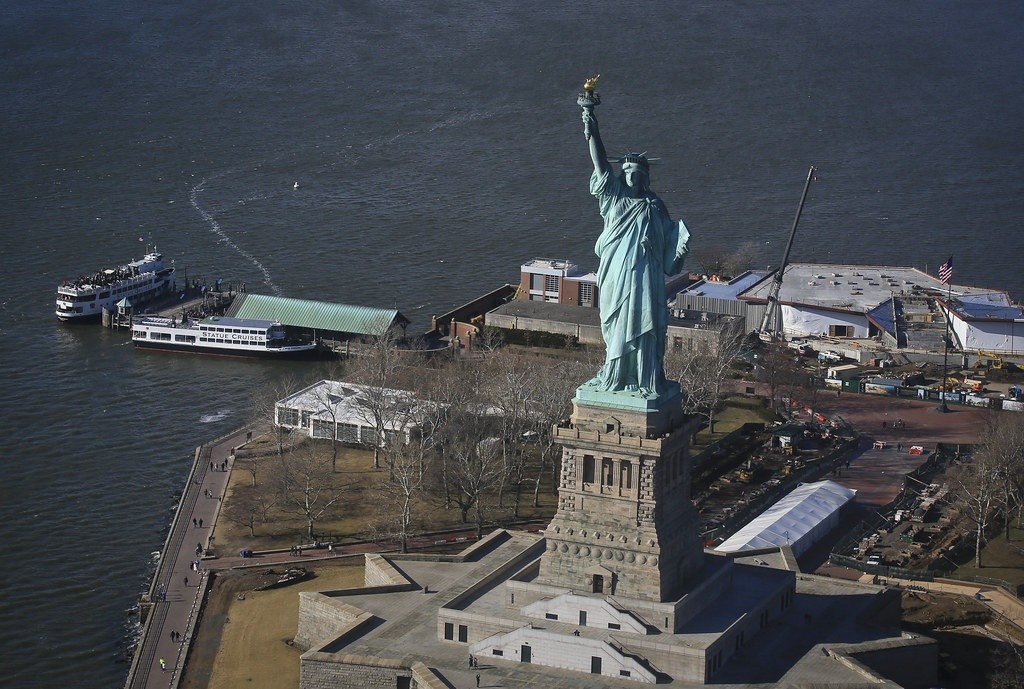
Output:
[867,556,880,565]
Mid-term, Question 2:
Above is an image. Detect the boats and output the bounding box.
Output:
[131,317,318,359]
[56,245,177,321]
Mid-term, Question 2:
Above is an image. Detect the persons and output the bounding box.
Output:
[883,419,905,431]
[204,489,212,498]
[158,592,166,600]
[582,112,690,395]
[192,277,232,295]
[837,389,840,397]
[313,539,336,554]
[187,310,226,319]
[897,442,904,452]
[210,447,234,471]
[184,576,188,586]
[240,549,252,558]
[169,629,181,643]
[189,542,202,572]
[159,657,166,673]
[290,545,302,557]
[831,460,849,476]
[59,265,138,301]
[192,518,203,528]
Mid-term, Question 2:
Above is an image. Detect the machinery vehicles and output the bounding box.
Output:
[754,163,818,343]
[974,348,1008,369]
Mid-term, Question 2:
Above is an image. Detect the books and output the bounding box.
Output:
[663,219,693,277]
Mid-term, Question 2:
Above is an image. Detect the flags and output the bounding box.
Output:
[938,257,953,285]
